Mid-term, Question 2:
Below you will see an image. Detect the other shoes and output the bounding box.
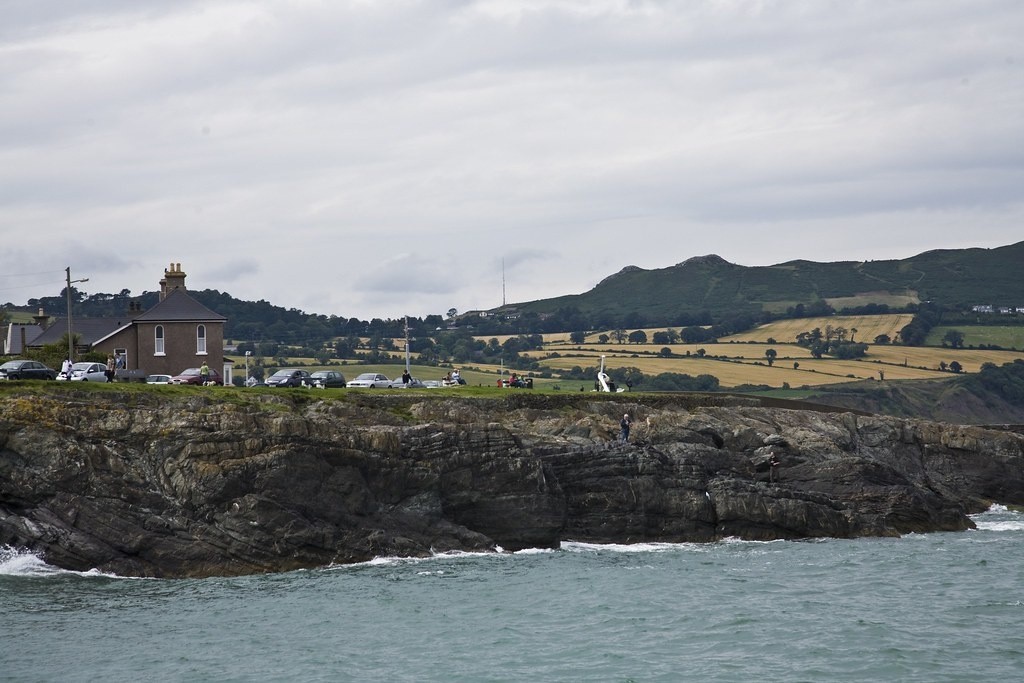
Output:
[106,381,112,384]
[112,379,118,382]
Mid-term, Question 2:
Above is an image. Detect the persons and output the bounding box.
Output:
[447,369,467,385]
[200,361,209,386]
[66,364,73,380]
[580,385,584,391]
[621,413,630,443]
[509,373,528,388]
[403,370,413,388]
[106,353,124,382]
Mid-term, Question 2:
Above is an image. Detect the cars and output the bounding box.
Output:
[0,360,57,381]
[310,371,347,389]
[346,373,393,388]
[393,376,427,389]
[169,368,224,385]
[148,374,172,384]
[422,380,444,388]
[58,362,118,382]
[232,375,269,388]
[265,368,315,388]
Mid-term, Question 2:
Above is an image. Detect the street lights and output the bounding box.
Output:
[67,278,89,363]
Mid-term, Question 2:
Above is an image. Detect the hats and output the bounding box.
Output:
[453,369,457,371]
[202,361,206,364]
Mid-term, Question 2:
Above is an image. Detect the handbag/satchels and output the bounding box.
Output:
[104,370,111,376]
